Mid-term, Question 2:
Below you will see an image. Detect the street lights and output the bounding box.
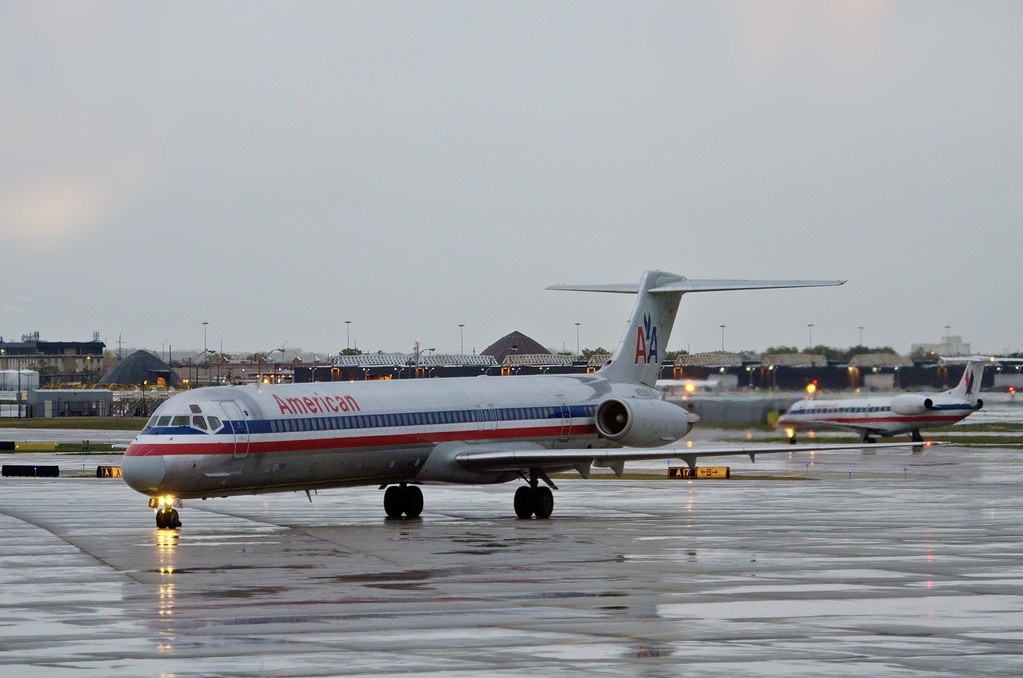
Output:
[858,326,864,346]
[489,347,519,376]
[344,321,351,353]
[944,325,951,354]
[808,323,815,348]
[408,348,435,379]
[201,321,209,365]
[459,324,465,355]
[720,325,726,352]
[574,323,581,360]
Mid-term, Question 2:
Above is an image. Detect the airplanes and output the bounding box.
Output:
[119,268,949,530]
[778,355,1023,450]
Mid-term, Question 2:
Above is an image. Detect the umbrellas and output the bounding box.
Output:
[96,349,182,416]
